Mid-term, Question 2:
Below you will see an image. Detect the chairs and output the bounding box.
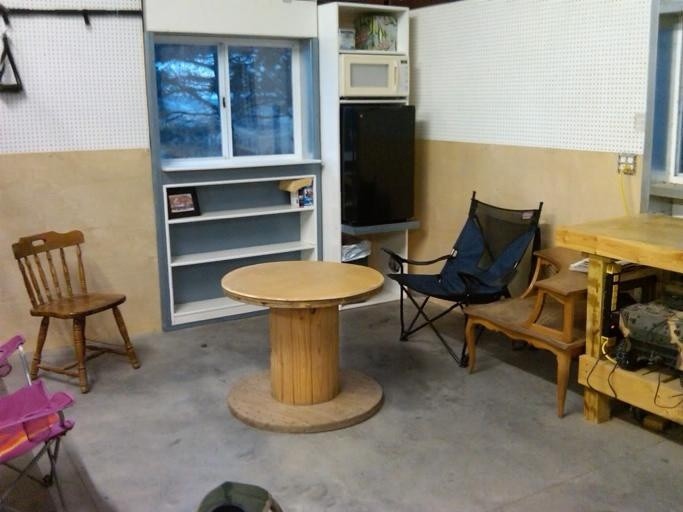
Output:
[0,334,78,512]
[11,231,140,393]
[381,191,544,366]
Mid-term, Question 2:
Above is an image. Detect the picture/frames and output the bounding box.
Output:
[167,186,201,220]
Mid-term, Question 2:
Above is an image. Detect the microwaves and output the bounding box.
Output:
[337,52,411,98]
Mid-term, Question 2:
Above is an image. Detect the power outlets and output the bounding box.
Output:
[617,152,637,176]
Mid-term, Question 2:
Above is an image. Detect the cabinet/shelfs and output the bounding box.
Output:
[317,2,420,311]
[162,174,318,326]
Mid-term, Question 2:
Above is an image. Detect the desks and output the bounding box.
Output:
[462,247,639,418]
[553,211,683,426]
